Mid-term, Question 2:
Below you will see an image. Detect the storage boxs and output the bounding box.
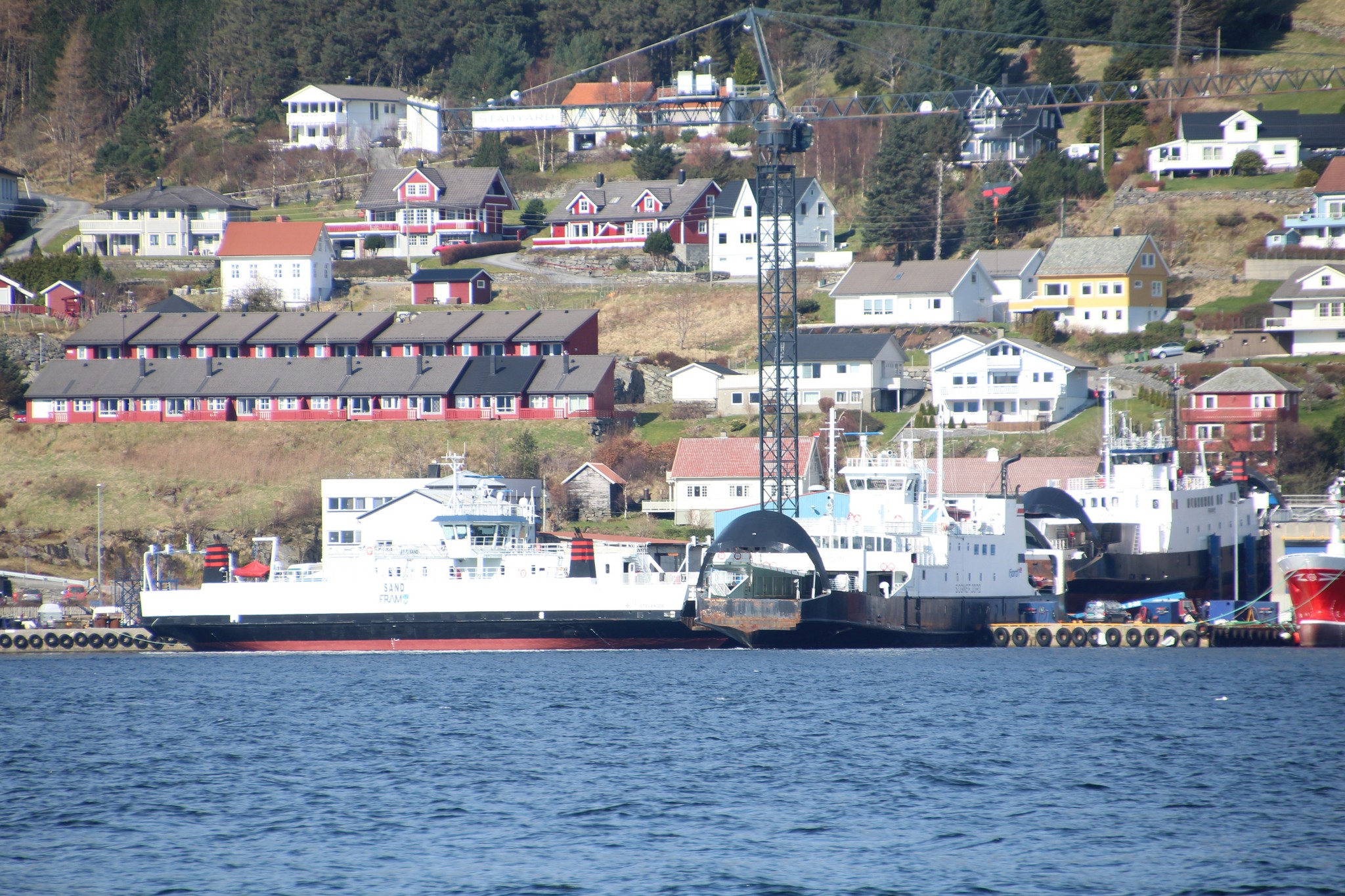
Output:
[1209,600,1245,624]
[1244,602,1278,623]
[1142,599,1179,623]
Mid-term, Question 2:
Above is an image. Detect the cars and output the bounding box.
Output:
[12,409,27,422]
[579,139,595,150]
[1151,342,1186,359]
[368,135,401,148]
[0,591,7,607]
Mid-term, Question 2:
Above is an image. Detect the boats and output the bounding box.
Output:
[135,438,747,655]
[1276,476,1345,648]
[693,362,1260,651]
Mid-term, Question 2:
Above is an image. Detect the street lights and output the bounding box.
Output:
[36,332,44,365]
[96,483,101,585]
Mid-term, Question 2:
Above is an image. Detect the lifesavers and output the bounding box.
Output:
[894,515,902,520]
[1105,628,1121,647]
[1180,629,1199,647]
[1036,628,1053,647]
[1195,623,1210,635]
[1138,606,1148,621]
[366,546,373,556]
[1096,478,1106,488]
[1012,627,1029,648]
[1126,628,1142,646]
[1209,627,1298,648]
[1055,628,1071,646]
[1145,608,1152,623]
[993,627,1010,646]
[1087,627,1102,647]
[1162,629,1180,648]
[520,569,527,578]
[1071,627,1088,647]
[0,632,178,649]
[734,552,742,560]
[853,514,861,522]
[1038,606,1046,614]
[1144,628,1159,647]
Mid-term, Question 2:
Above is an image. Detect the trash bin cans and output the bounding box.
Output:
[1137,351,1143,361]
[820,279,827,286]
[1124,353,1130,364]
[1142,350,1149,361]
[1129,352,1135,363]
[193,248,199,255]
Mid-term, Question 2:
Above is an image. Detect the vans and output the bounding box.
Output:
[62,585,87,605]
[1084,600,1131,624]
[1058,143,1101,162]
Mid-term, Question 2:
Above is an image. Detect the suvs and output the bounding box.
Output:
[21,588,43,607]
